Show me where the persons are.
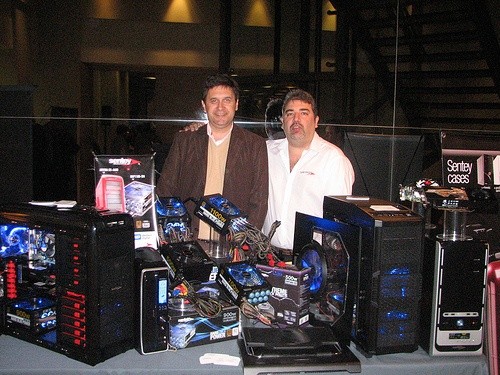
[110,124,128,155]
[324,126,372,196]
[155,73,269,251]
[265,98,287,140]
[179,89,355,262]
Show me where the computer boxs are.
[135,247,169,356]
[323,194,426,359]
[0,203,137,366]
[423,229,489,358]
